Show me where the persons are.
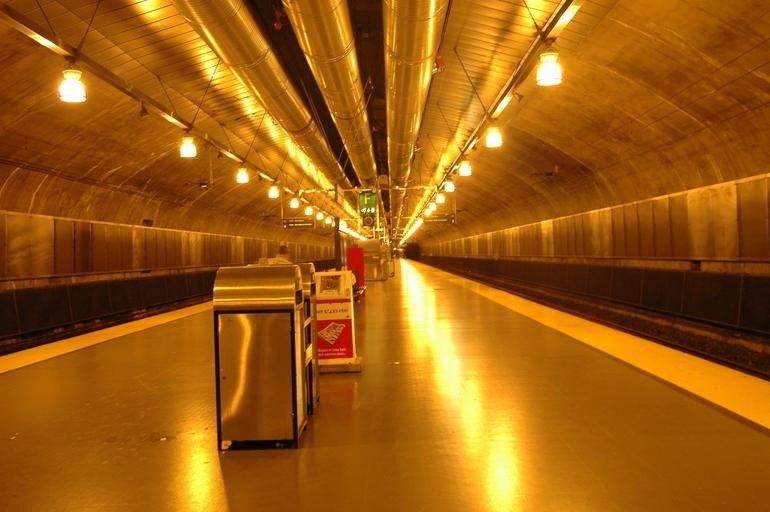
[267,246,293,264]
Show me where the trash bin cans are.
[213,263,320,451]
